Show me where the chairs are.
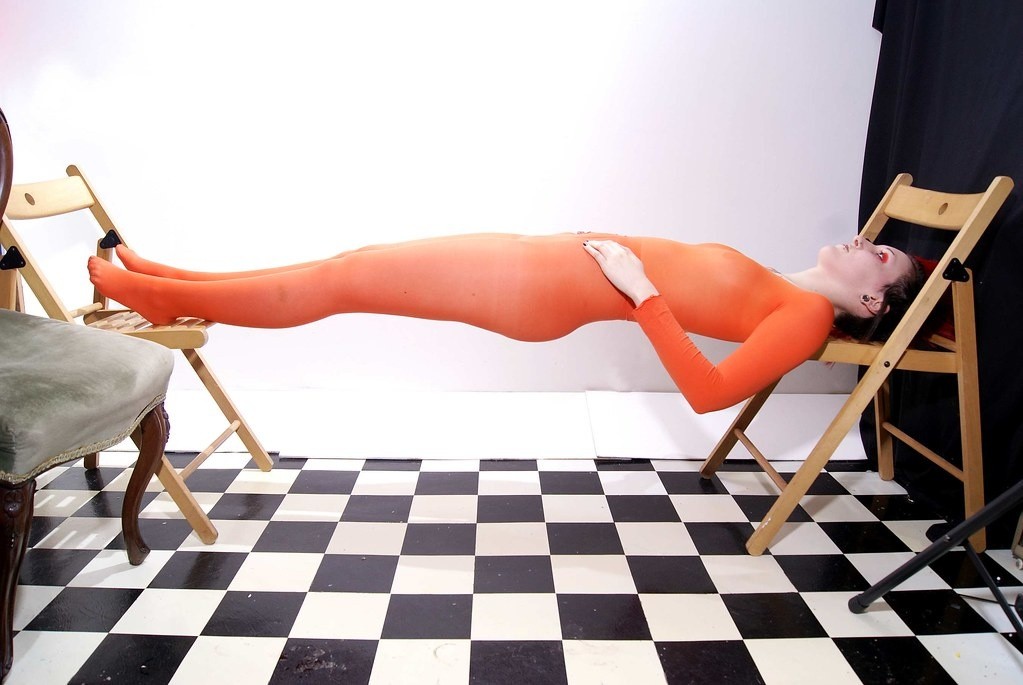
[0,106,173,685]
[702,174,1015,558]
[0,163,272,546]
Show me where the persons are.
[88,232,928,415]
